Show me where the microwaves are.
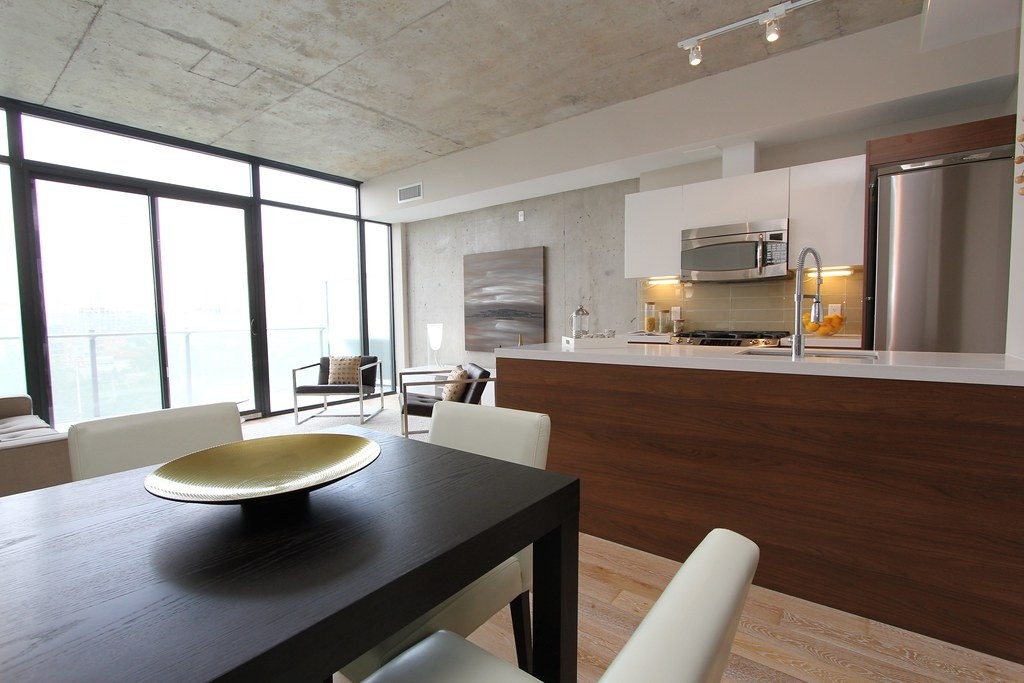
[680,217,789,283]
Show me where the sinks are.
[815,353,879,359]
[741,350,815,357]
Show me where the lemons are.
[801,313,844,335]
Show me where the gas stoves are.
[670,327,791,347]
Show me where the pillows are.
[441,365,468,401]
[328,356,361,384]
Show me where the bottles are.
[659,309,669,332]
[645,301,655,333]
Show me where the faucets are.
[787,245,824,358]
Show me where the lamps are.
[689,40,705,67]
[765,16,780,42]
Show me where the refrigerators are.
[871,141,1017,355]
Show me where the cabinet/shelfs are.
[625,153,866,278]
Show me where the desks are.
[0,424,580,683]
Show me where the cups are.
[673,318,684,333]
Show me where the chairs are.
[291,355,383,425]
[356,527,762,683]
[0,394,67,498]
[344,401,552,673]
[67,401,245,482]
[398,363,491,438]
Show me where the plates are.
[142,433,382,506]
